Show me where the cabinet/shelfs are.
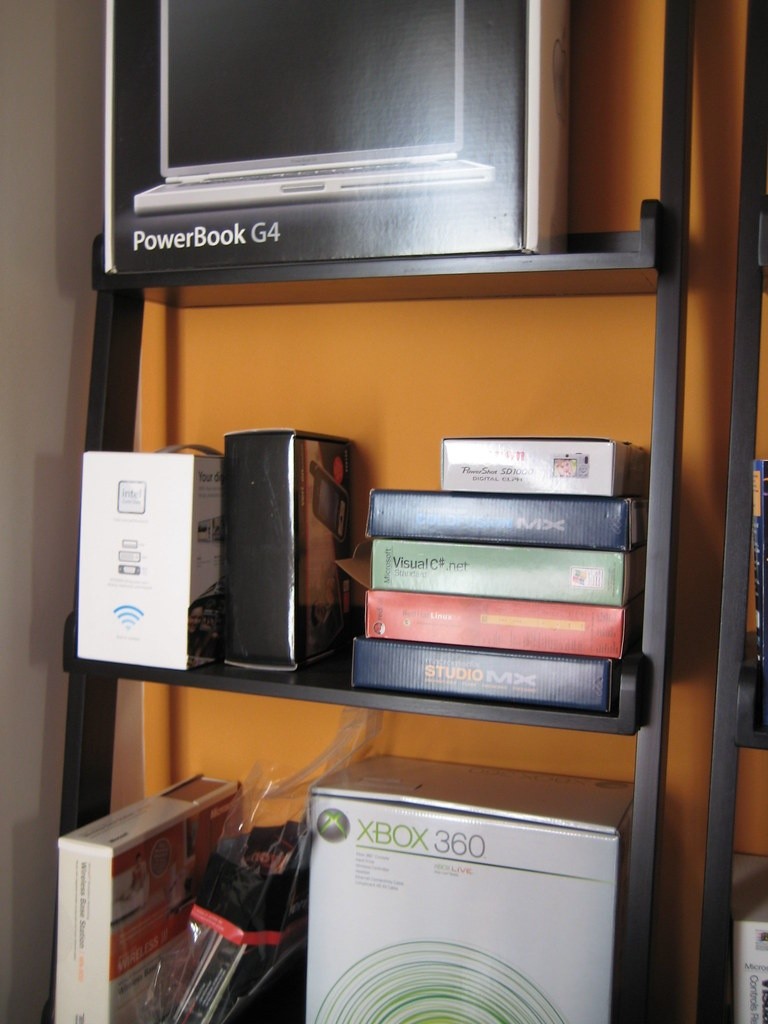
[43,1,695,1024]
[700,0,767,1024]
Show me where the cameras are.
[551,452,591,480]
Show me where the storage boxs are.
[306,754,633,1024]
[222,428,351,673]
[731,852,768,1024]
[77,446,223,670]
[352,436,650,714]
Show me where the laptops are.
[134,0,495,218]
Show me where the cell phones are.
[309,460,349,542]
